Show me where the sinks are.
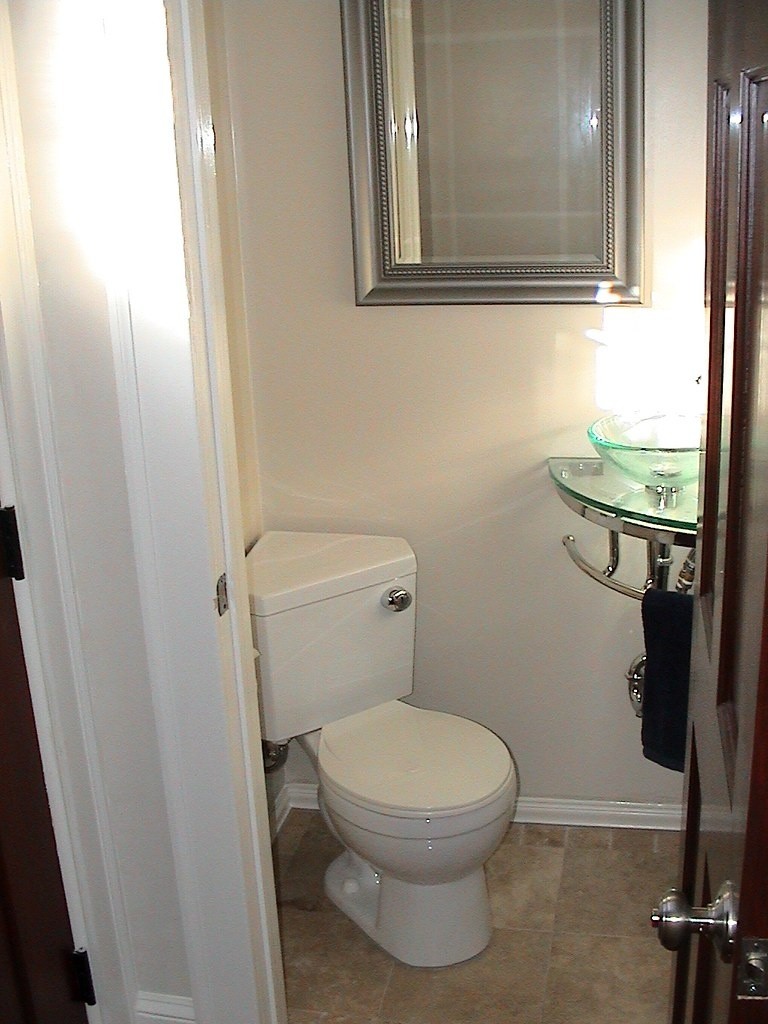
[585,407,706,490]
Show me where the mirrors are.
[339,0,645,308]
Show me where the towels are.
[639,586,696,774]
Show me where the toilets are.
[241,529,521,970]
